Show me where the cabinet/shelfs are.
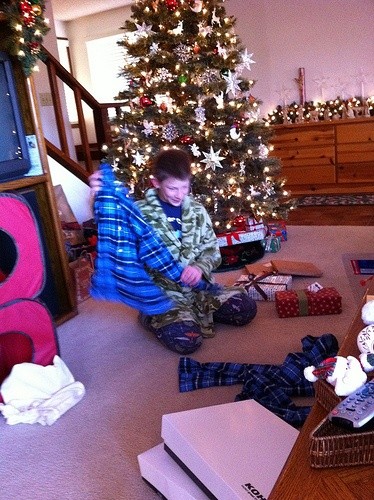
[272,118,374,198]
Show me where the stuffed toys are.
[303,355,367,398]
[357,300,374,374]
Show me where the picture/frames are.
[0,173,80,329]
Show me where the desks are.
[266,273,374,500]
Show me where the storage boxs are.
[160,399,301,500]
[275,287,343,318]
[233,274,293,303]
[217,224,266,247]
[137,441,209,500]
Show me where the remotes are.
[328,376,374,428]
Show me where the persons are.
[89,150,257,356]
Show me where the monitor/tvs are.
[0,50,32,182]
